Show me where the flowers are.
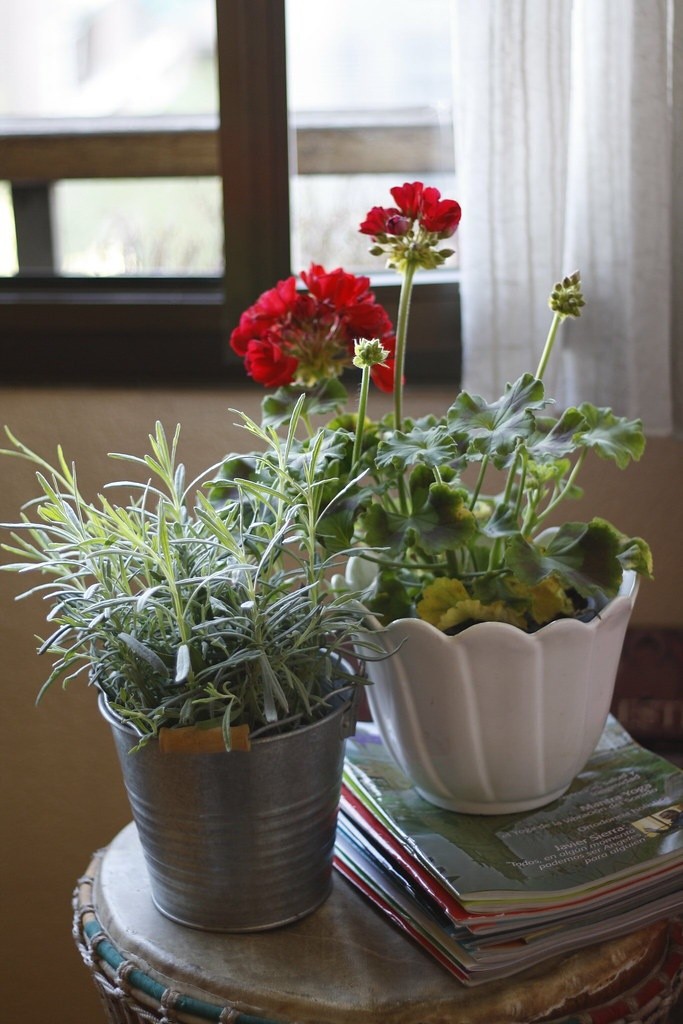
[202,176,658,625]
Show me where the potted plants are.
[0,388,411,933]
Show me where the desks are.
[69,821,683,1024]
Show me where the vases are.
[328,527,638,816]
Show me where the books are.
[331,712,683,988]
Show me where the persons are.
[644,810,683,834]
